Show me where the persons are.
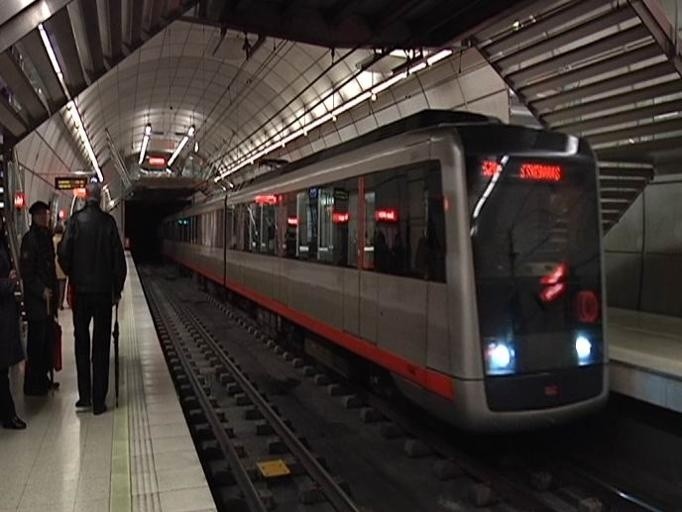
[62,219,73,308]
[51,224,66,312]
[18,200,59,397]
[57,181,127,416]
[0,214,27,431]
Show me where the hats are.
[29,201,50,213]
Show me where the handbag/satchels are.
[53,321,61,371]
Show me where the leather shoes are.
[3,417,26,429]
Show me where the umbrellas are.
[111,302,122,410]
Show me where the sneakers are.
[76,400,91,406]
[94,404,106,414]
[24,376,59,395]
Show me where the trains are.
[160,107,613,433]
